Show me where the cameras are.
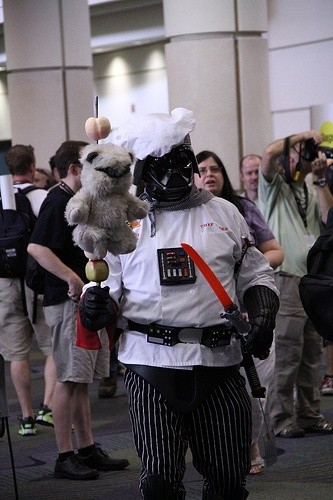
[305,138,333,162]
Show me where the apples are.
[85,260,109,281]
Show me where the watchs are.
[313,177,326,187]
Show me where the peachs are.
[85,117,111,141]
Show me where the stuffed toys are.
[65,141,151,264]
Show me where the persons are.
[83,107,283,500]
[23,142,131,482]
[32,169,55,191]
[192,149,284,476]
[236,154,261,205]
[0,144,75,437]
[258,130,333,438]
[48,149,63,188]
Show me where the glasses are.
[198,166,223,174]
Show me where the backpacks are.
[0,185,43,277]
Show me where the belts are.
[125,320,237,343]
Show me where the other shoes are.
[76,448,130,471]
[54,455,100,480]
[320,375,333,395]
[276,424,305,439]
[309,419,333,432]
[96,380,118,399]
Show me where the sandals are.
[250,439,265,474]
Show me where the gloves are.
[243,326,275,360]
[83,284,113,323]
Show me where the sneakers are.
[37,404,76,432]
[17,415,38,437]
